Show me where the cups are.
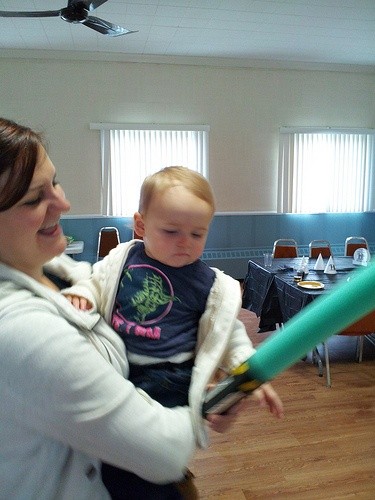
[263,254,273,267]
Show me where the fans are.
[0,0,139,38]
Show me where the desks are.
[241,257,375,376]
[64,241,84,260]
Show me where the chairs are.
[309,240,332,258]
[97,227,120,262]
[345,237,369,257]
[312,306,375,387]
[272,239,298,258]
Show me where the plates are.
[297,280,325,289]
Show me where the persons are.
[0,118,246,500]
[59,166,283,500]
